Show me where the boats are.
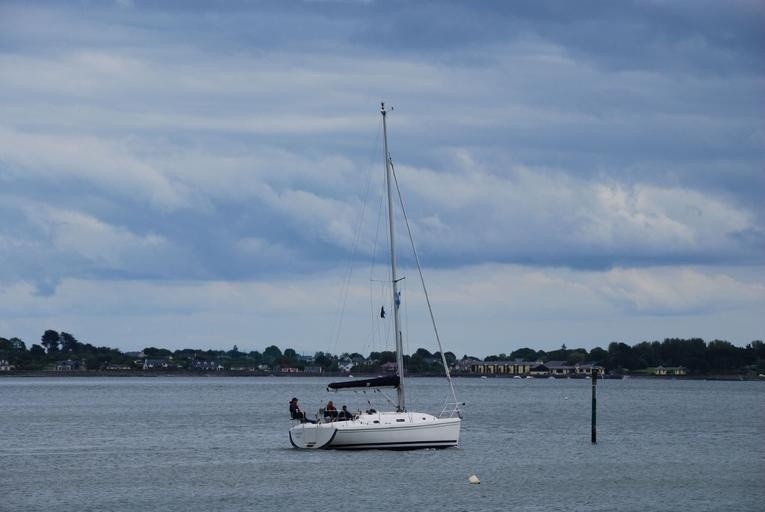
[327,374,401,390]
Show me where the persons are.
[336,404,353,420]
[323,400,338,421]
[288,397,316,424]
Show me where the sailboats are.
[285,98,469,452]
